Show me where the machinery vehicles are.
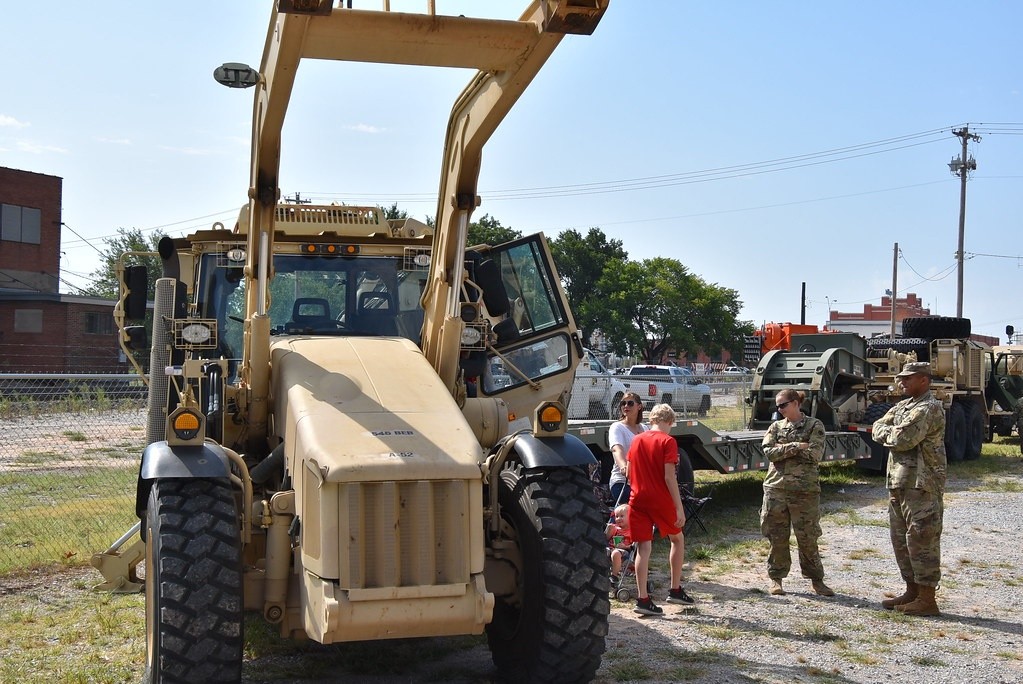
[569,316,1023,518]
[91,0,614,684]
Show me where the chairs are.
[293,298,337,328]
[359,292,397,336]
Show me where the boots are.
[882,582,940,616]
[771,578,784,594]
[812,579,835,596]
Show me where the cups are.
[612,535,623,548]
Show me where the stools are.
[677,481,721,535]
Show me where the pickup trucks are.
[618,364,713,418]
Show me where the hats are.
[896,362,932,377]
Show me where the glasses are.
[776,400,793,411]
[620,400,639,407]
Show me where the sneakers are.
[633,595,663,616]
[666,585,694,605]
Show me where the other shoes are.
[609,573,622,583]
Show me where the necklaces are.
[628,423,640,434]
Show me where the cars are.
[722,366,748,374]
[567,348,628,420]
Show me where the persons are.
[626,403,694,614]
[872,362,947,617]
[608,393,652,574]
[758,390,835,596]
[604,504,633,583]
